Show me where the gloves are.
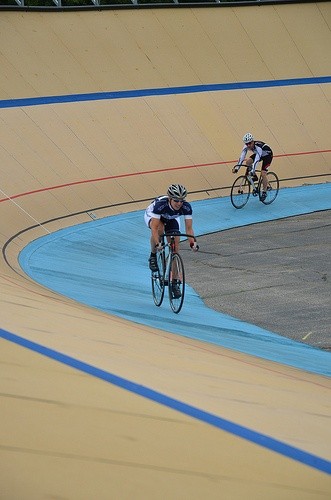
[155,243,164,251]
[190,241,199,253]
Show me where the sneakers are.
[173,285,181,298]
[148,255,159,272]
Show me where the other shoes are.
[260,192,267,201]
[248,176,258,184]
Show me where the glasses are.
[245,141,253,145]
[172,199,186,203]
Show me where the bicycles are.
[230,164,280,210]
[151,222,200,313]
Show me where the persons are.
[231,134,274,201]
[145,184,199,299]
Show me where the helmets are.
[242,133,254,144]
[167,184,188,201]
